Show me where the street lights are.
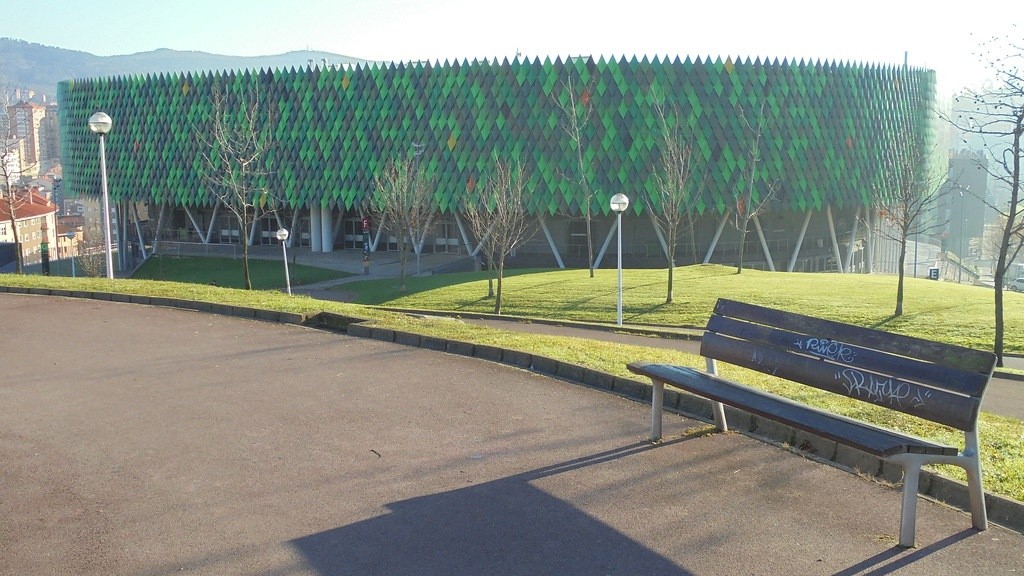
[88,112,112,277]
[610,192,629,325]
[276,228,291,296]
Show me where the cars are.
[1008,278,1024,292]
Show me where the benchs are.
[627,297,997,548]
